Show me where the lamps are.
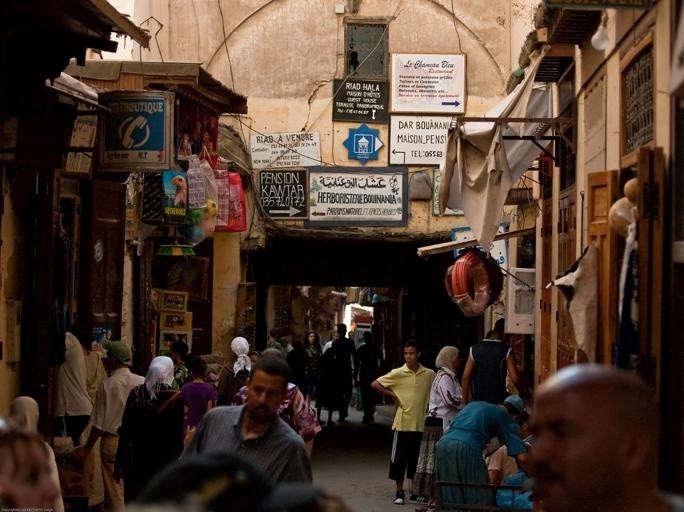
[590,9,611,51]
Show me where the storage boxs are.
[159,312,193,330]
[160,289,189,312]
[158,330,192,354]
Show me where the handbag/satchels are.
[140,153,247,232]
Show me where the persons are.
[371,318,684,510]
[176,111,216,161]
[1,323,343,512]
[303,324,384,425]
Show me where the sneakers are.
[409,495,428,504]
[394,490,404,504]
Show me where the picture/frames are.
[304,166,408,226]
[388,113,457,167]
[451,223,507,268]
[387,51,467,115]
[260,167,311,220]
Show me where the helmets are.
[103,339,128,361]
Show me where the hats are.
[114,347,117,348]
[506,395,524,414]
